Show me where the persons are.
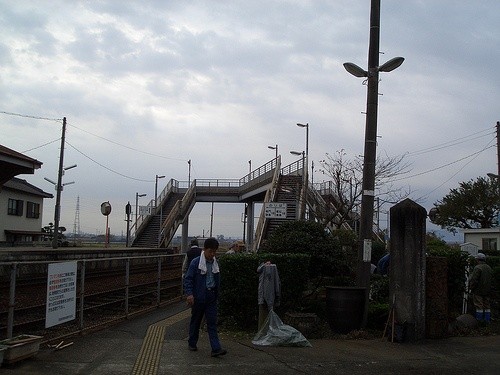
[182,238,203,306]
[226,241,246,254]
[469,253,493,321]
[184,238,228,357]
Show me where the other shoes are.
[188,344,198,352]
[210,349,227,357]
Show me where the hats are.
[475,253,486,261]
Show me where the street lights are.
[154,175,165,206]
[135,192,146,231]
[269,145,277,170]
[343,57,405,335]
[297,122,309,186]
[290,150,305,184]
